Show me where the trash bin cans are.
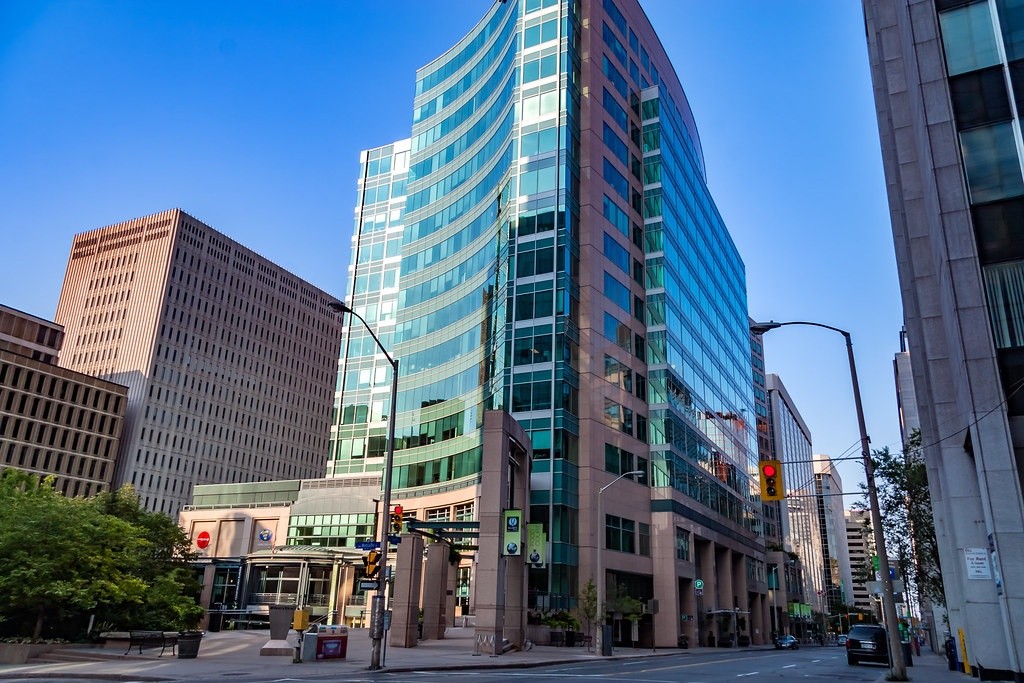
[901,640,913,667]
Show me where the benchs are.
[549,632,565,647]
[576,633,593,648]
[124,630,178,658]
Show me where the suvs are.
[846,622,893,667]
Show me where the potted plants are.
[565,618,580,647]
[173,605,204,658]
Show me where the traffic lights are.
[360,554,369,578]
[393,506,403,531]
[369,551,383,575]
[759,460,784,501]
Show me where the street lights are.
[596,471,644,655]
[327,299,399,669]
[753,322,912,682]
[821,588,836,625]
[773,560,794,637]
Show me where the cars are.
[775,635,799,650]
[838,637,847,646]
